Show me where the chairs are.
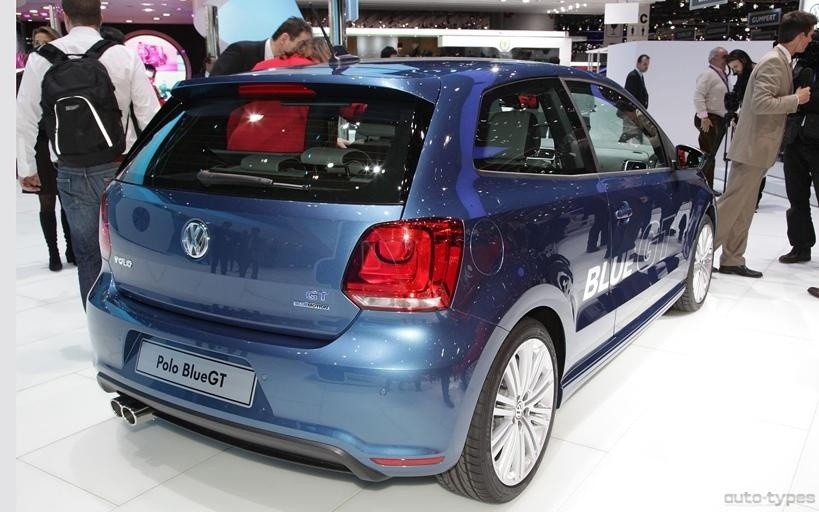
[348,122,394,160]
[476,113,559,176]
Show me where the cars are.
[85,56,718,505]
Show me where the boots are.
[60,210,79,267]
[39,211,62,271]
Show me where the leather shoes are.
[712,189,721,197]
[808,287,819,298]
[779,245,811,263]
[712,263,763,277]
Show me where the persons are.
[16,0,161,315]
[100,23,126,47]
[379,46,398,58]
[692,46,732,199]
[203,220,276,279]
[16,26,77,272]
[616,53,652,144]
[777,27,818,264]
[222,39,369,154]
[725,48,768,215]
[207,16,365,153]
[583,180,681,263]
[713,9,819,279]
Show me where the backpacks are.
[34,39,134,167]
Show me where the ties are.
[642,74,645,89]
